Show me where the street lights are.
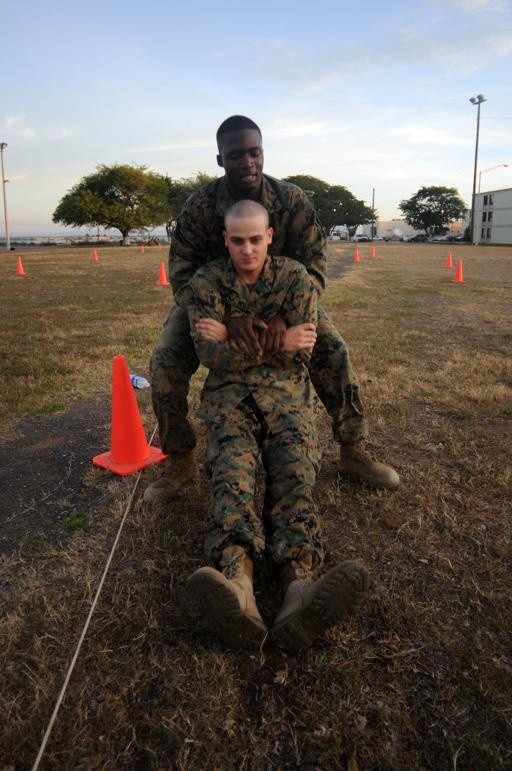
[477,164,509,192]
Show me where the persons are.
[141,114,401,506]
[186,198,371,657]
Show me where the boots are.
[143,447,198,504]
[273,560,369,654]
[187,544,269,645]
[337,442,401,491]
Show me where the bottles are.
[129,374,150,389]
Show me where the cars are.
[454,234,464,242]
[328,229,386,242]
[431,235,450,241]
[402,233,429,245]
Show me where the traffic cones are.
[139,244,146,254]
[449,257,465,284]
[368,244,377,257]
[15,256,27,277]
[446,250,454,270]
[157,244,162,251]
[351,247,360,264]
[91,249,100,262]
[154,260,171,287]
[90,351,168,477]
